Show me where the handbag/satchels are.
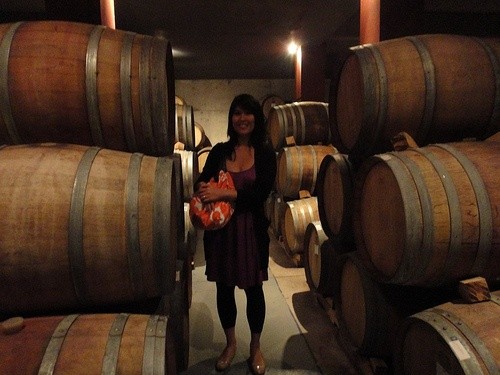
[189,143,237,230]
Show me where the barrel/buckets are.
[0,19,215,375]
[256,33,499,374]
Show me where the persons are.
[194,94,277,375]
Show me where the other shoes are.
[216,340,237,371]
[250,342,266,374]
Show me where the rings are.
[204,195,205,197]
